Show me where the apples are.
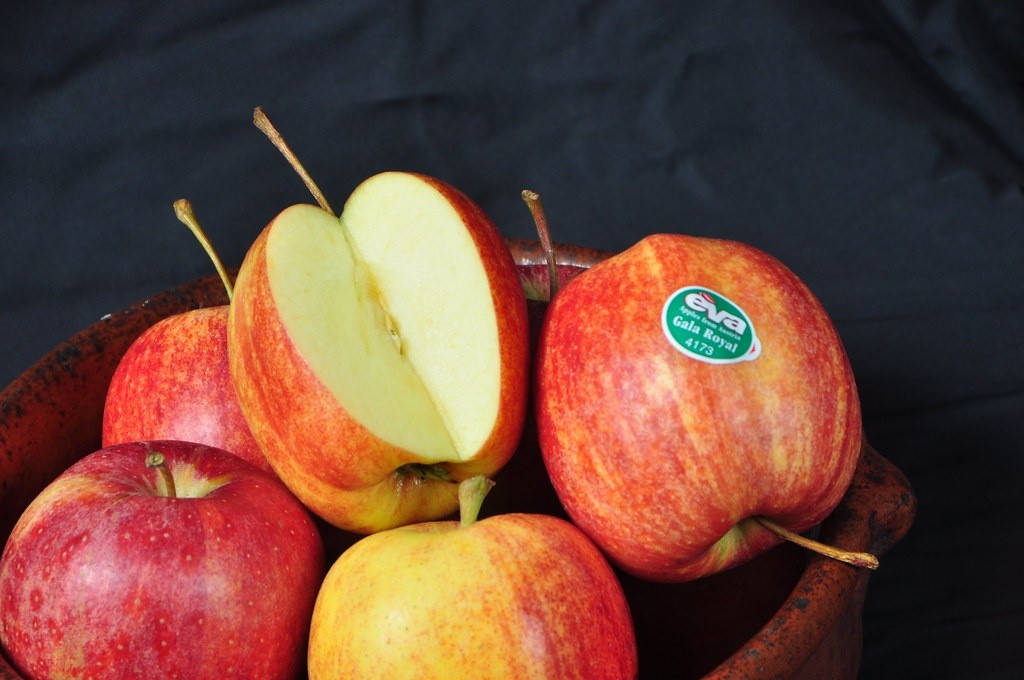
[0,107,881,680]
